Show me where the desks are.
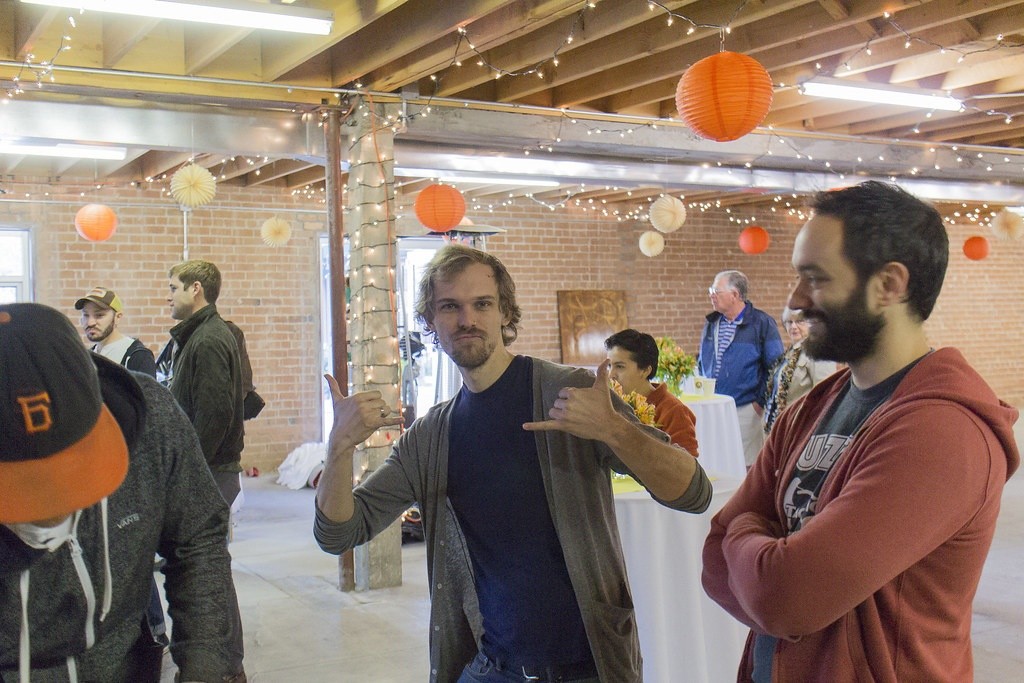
[671,394,748,476]
[615,475,751,683]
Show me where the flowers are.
[605,378,655,488]
[653,336,695,398]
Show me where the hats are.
[0,302,129,525]
[75,286,122,312]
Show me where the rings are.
[380,408,385,418]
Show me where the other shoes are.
[173,667,248,683]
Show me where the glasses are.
[708,286,735,295]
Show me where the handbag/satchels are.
[400,330,424,366]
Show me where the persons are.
[0,302,247,683]
[701,181,1020,683]
[74,260,266,655]
[697,270,835,472]
[604,329,700,458]
[313,244,713,683]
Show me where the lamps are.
[797,75,962,112]
[20,0,333,37]
